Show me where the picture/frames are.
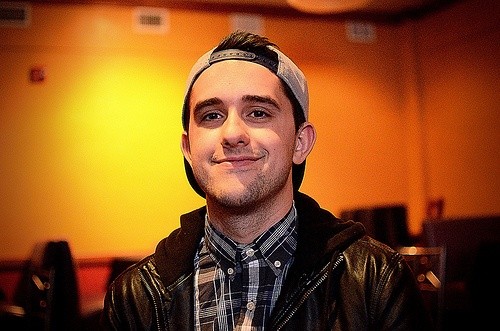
[390,246,446,292]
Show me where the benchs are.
[0,238,108,331]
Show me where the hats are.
[182,45,309,199]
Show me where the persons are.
[99,33,427,331]
